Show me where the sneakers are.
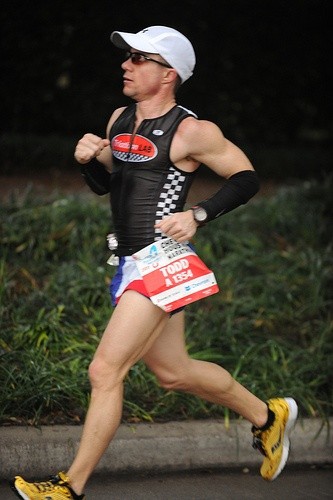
[11,470,84,500]
[252,396,298,481]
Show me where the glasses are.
[123,52,173,67]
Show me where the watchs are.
[190,205,207,226]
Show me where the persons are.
[0,26,298,500]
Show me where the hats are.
[109,25,196,83]
